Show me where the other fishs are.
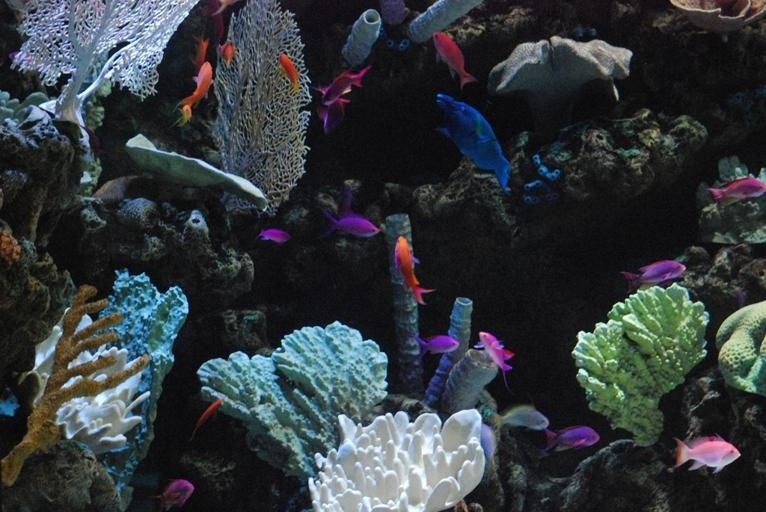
[169,0,237,135]
